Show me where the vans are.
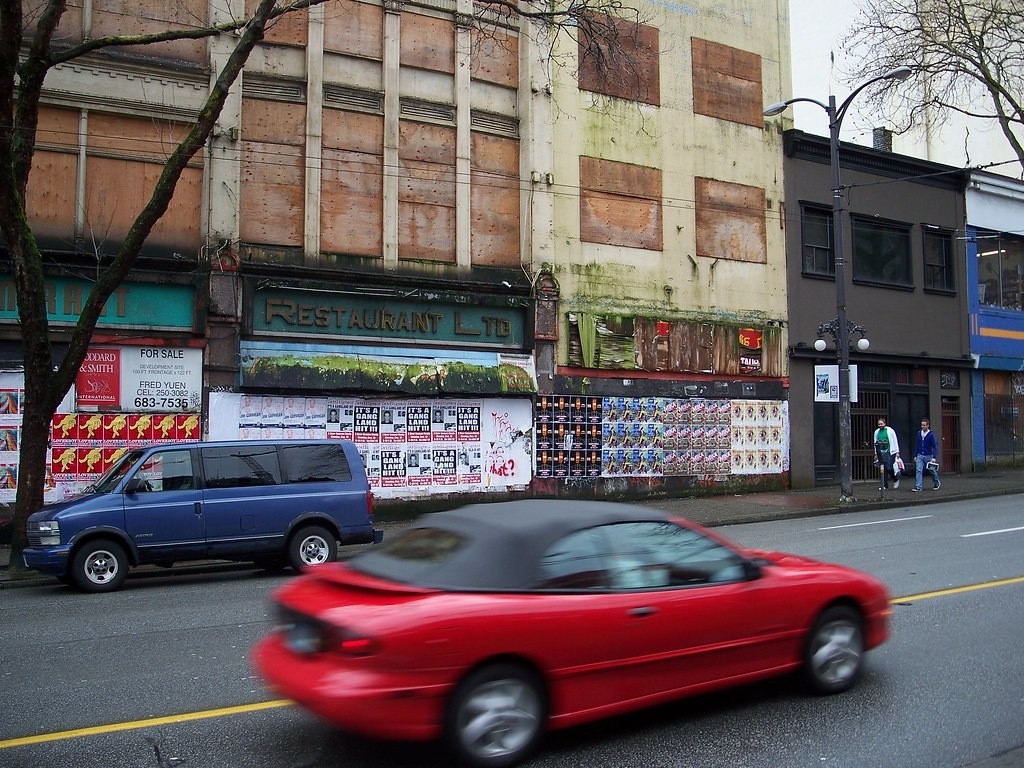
[23,438,384,594]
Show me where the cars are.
[256,498,891,768]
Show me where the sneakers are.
[893,479,899,488]
[911,487,923,492]
[932,479,941,490]
[878,486,887,490]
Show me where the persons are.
[911,418,942,492]
[874,418,902,490]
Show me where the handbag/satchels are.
[893,456,905,476]
[925,461,939,471]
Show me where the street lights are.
[762,65,911,502]
